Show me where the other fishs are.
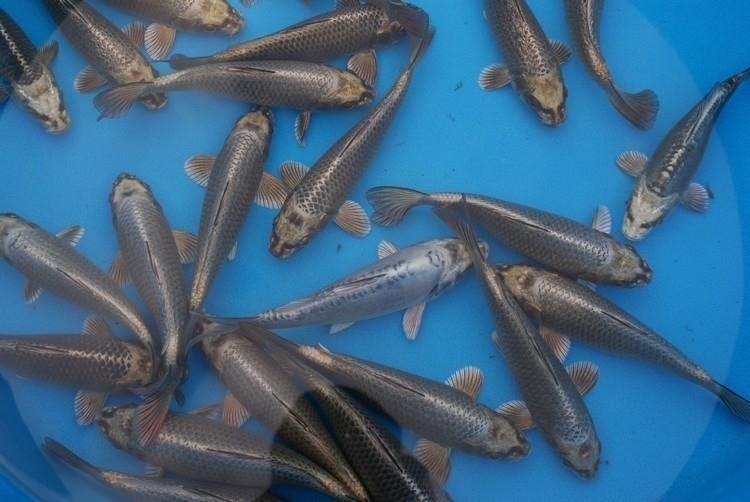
[1,1,746,502]
